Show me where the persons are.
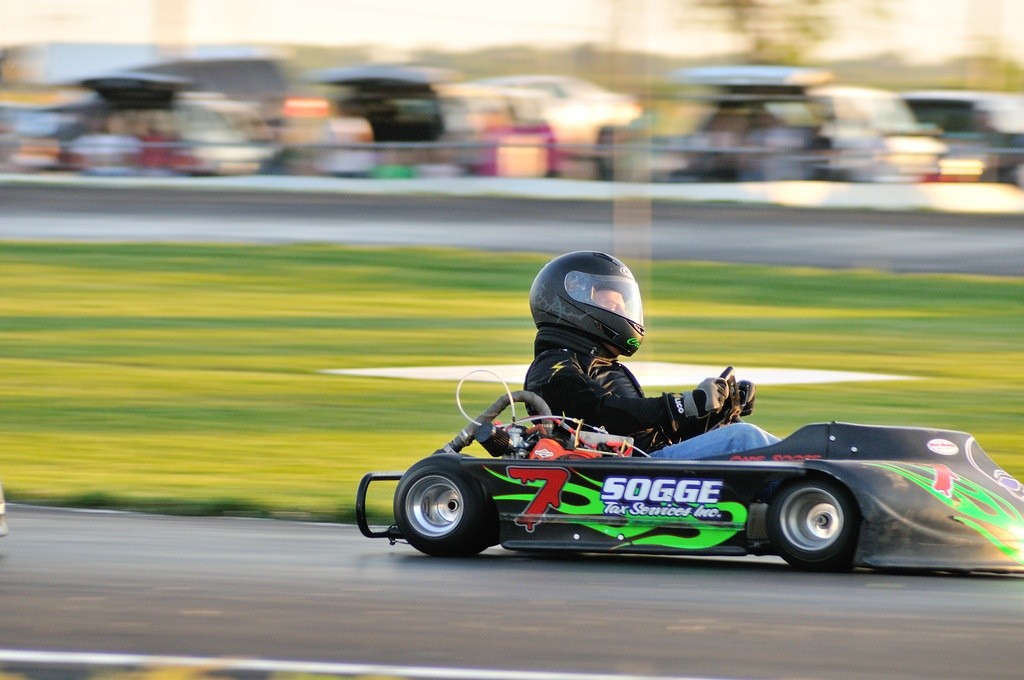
[523,249,785,460]
[0,100,1024,191]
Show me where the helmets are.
[529,250,646,354]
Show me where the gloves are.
[731,379,757,417]
[680,376,728,418]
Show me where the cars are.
[0,48,1024,186]
